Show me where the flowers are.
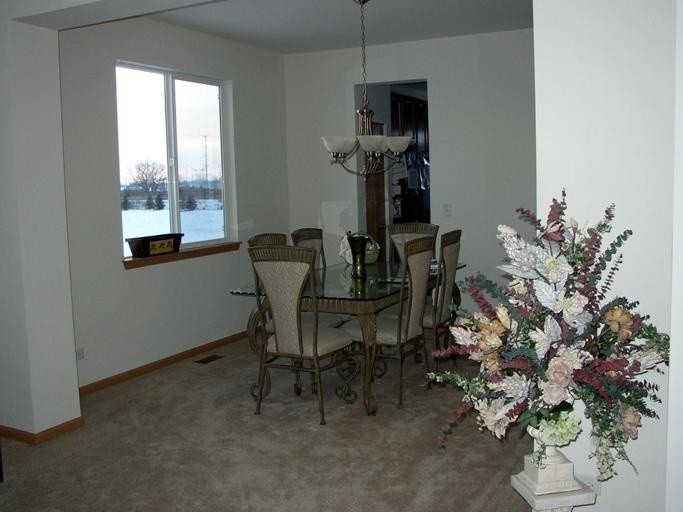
[418,187,670,483]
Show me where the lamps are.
[320,1,411,178]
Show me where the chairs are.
[245,231,345,395]
[384,223,442,372]
[288,227,358,327]
[376,228,464,388]
[338,234,437,409]
[245,243,358,425]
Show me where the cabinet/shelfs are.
[390,92,430,224]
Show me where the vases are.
[124,233,184,258]
[512,425,583,498]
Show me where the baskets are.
[350,232,380,265]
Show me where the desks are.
[230,257,468,417]
